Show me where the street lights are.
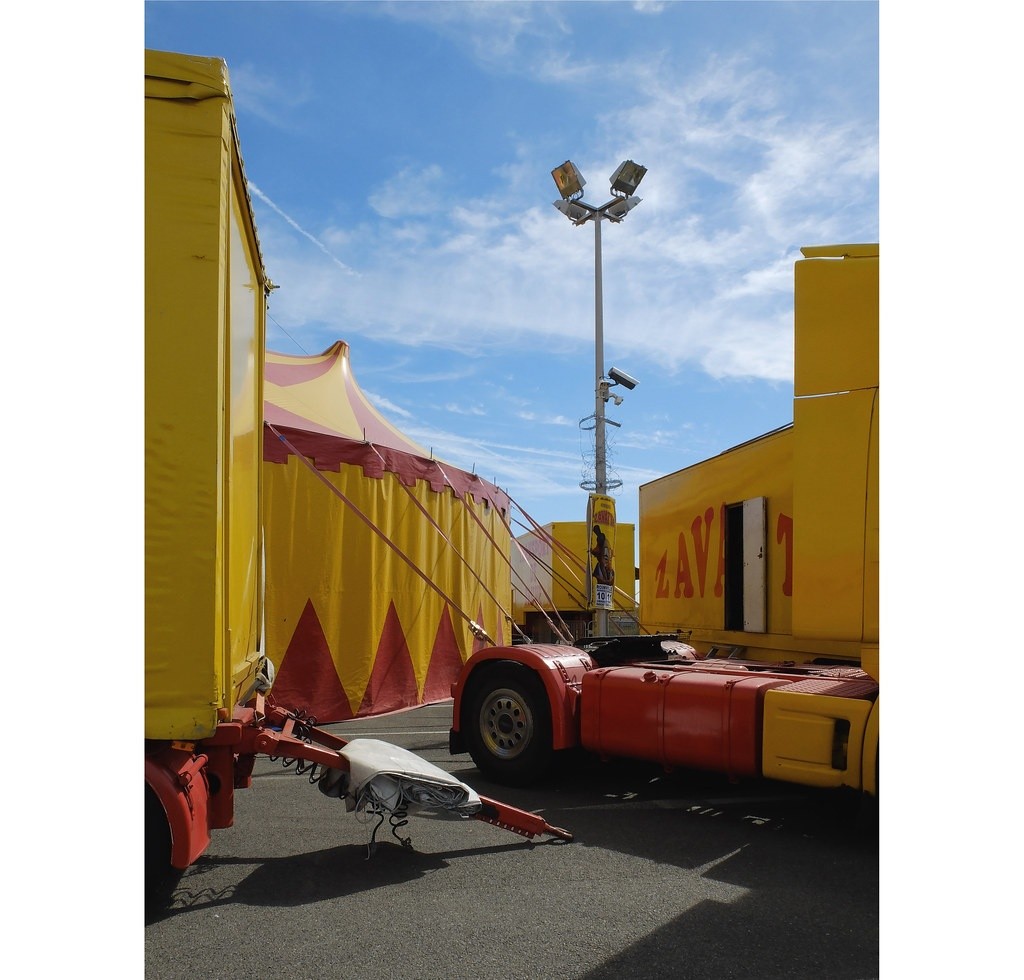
[551,159,648,645]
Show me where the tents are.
[261,340,513,729]
